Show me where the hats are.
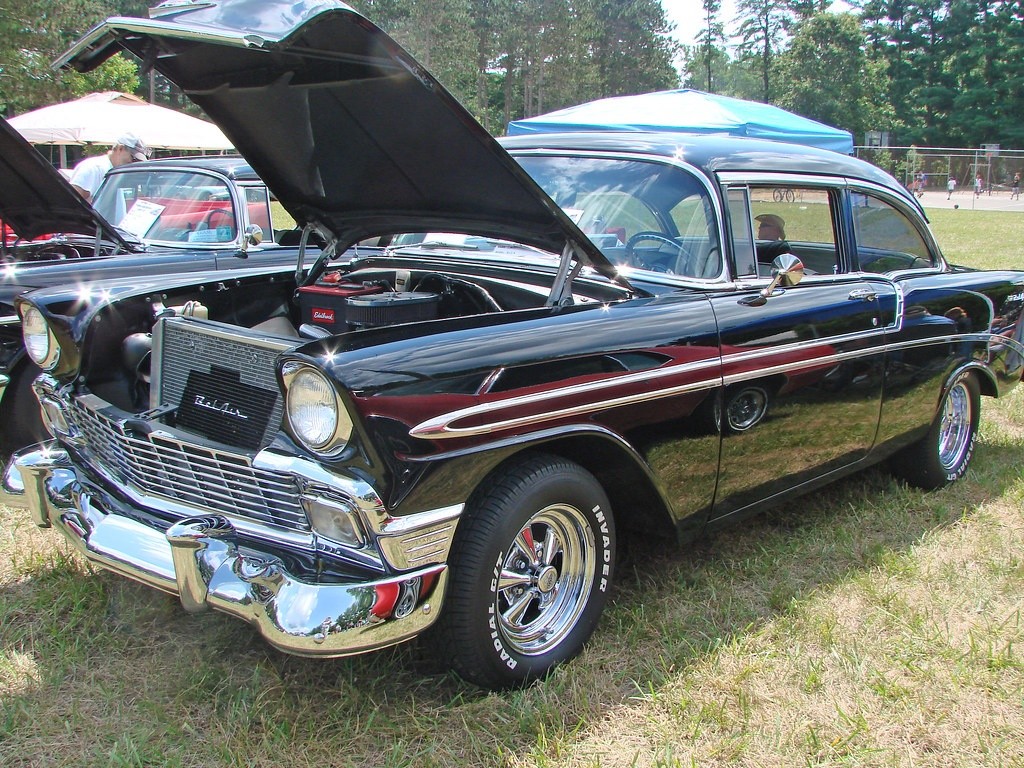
[754,213,785,229]
[117,132,152,162]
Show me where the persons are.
[975,175,982,197]
[915,169,927,198]
[754,213,788,242]
[948,176,957,201]
[69,131,147,228]
[1010,174,1020,201]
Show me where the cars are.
[1,175,269,248]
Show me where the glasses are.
[759,222,774,227]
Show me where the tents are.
[509,88,854,156]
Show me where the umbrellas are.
[7,91,235,151]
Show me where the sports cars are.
[0,113,626,387]
[0,0,1024,689]
